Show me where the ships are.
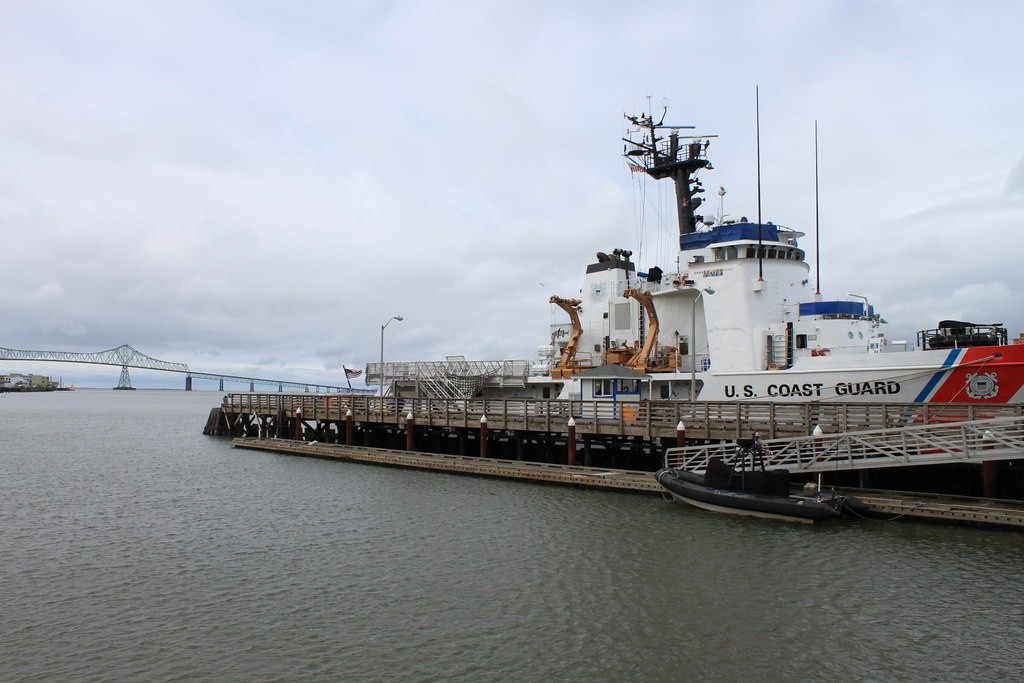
[363,87,1024,455]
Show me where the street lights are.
[379,314,404,397]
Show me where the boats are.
[654,433,869,528]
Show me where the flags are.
[627,161,647,173]
[344,368,363,379]
